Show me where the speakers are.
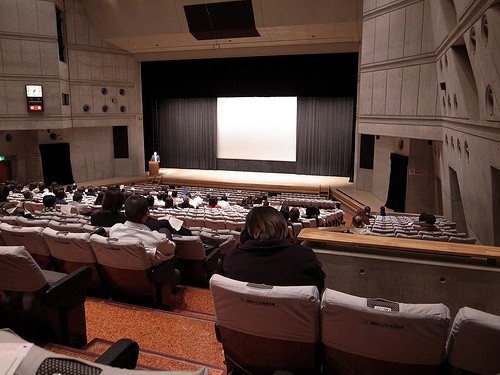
[428,140,432,145]
[440,82,446,90]
[376,135,380,139]
[47,129,50,133]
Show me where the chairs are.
[0,182,500,375]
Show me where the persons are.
[344,206,376,233]
[331,203,346,224]
[223,206,326,293]
[412,214,441,231]
[375,206,388,216]
[413,213,428,225]
[151,152,160,162]
[0,179,320,295]
